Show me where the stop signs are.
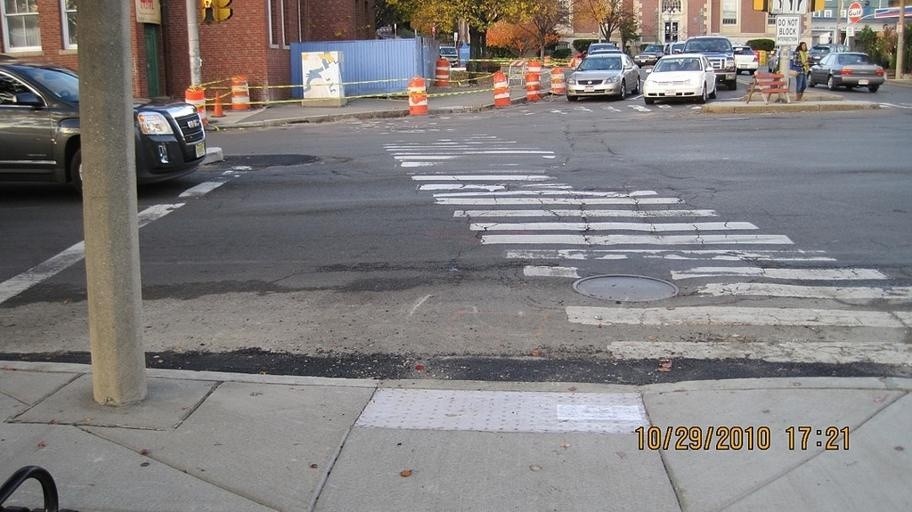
[848,2,863,24]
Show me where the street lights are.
[394,23,397,37]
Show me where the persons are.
[790,40,811,101]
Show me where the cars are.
[664,40,685,55]
[768,49,795,72]
[642,53,717,104]
[805,52,887,92]
[566,53,642,101]
[634,43,670,70]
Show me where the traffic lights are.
[213,0,232,22]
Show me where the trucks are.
[439,46,459,66]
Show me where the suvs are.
[673,35,739,90]
[590,48,624,55]
[732,45,759,75]
[588,41,617,56]
[807,43,849,66]
[1,62,206,187]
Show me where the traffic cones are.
[211,90,227,120]
